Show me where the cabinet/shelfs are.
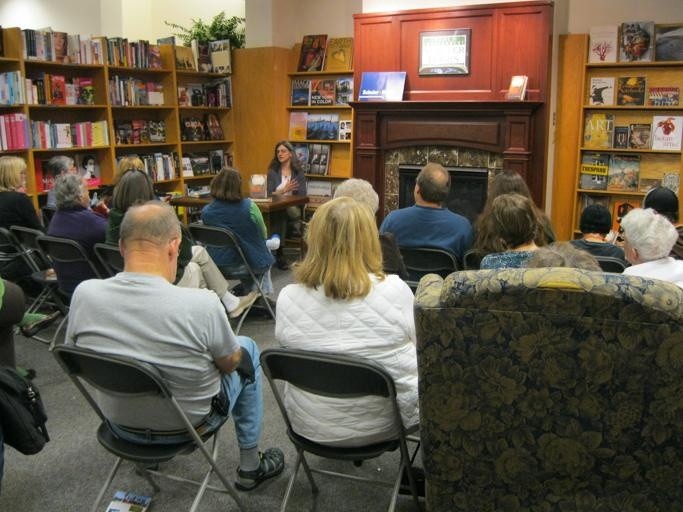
[287,38,354,181]
[0,27,236,233]
[550,34,682,246]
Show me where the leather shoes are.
[228,291,256,321]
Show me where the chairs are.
[588,254,628,275]
[91,237,130,284]
[395,246,460,285]
[49,340,254,511]
[257,344,425,511]
[0,224,44,341]
[462,249,494,272]
[418,268,682,512]
[189,221,278,338]
[28,234,104,348]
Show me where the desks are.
[167,186,314,272]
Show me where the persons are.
[479,193,544,270]
[93,154,147,216]
[81,156,96,182]
[379,162,474,272]
[268,140,308,271]
[0,277,61,380]
[474,173,555,254]
[275,197,421,449]
[333,177,378,221]
[47,156,81,211]
[622,206,682,290]
[63,201,285,493]
[0,156,46,278]
[643,187,683,261]
[568,205,632,275]
[105,169,258,319]
[200,167,280,300]
[522,240,602,274]
[45,175,114,296]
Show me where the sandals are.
[233,447,286,490]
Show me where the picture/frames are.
[418,28,472,76]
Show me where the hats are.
[642,184,680,214]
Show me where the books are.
[25,70,96,104]
[178,76,233,107]
[312,78,337,106]
[191,39,232,75]
[506,75,529,101]
[23,27,104,64]
[578,152,610,190]
[576,194,610,230]
[74,153,101,187]
[249,173,268,200]
[620,20,656,62]
[175,45,195,71]
[652,115,683,151]
[105,37,163,68]
[0,113,31,151]
[0,70,25,105]
[34,153,44,192]
[614,125,629,149]
[638,177,663,193]
[629,123,652,149]
[617,75,646,107]
[292,143,309,174]
[185,183,210,199]
[179,112,225,141]
[288,112,308,140]
[290,79,312,106]
[607,153,640,192]
[583,112,614,149]
[296,34,328,72]
[612,199,641,247]
[110,75,166,105]
[116,150,181,181]
[661,165,679,194]
[157,36,176,68]
[649,86,680,107]
[335,77,354,106]
[247,196,272,203]
[183,148,234,177]
[637,154,679,195]
[654,24,682,61]
[587,24,621,63]
[307,144,332,176]
[32,119,109,149]
[589,76,615,107]
[324,37,355,71]
[113,118,167,145]
